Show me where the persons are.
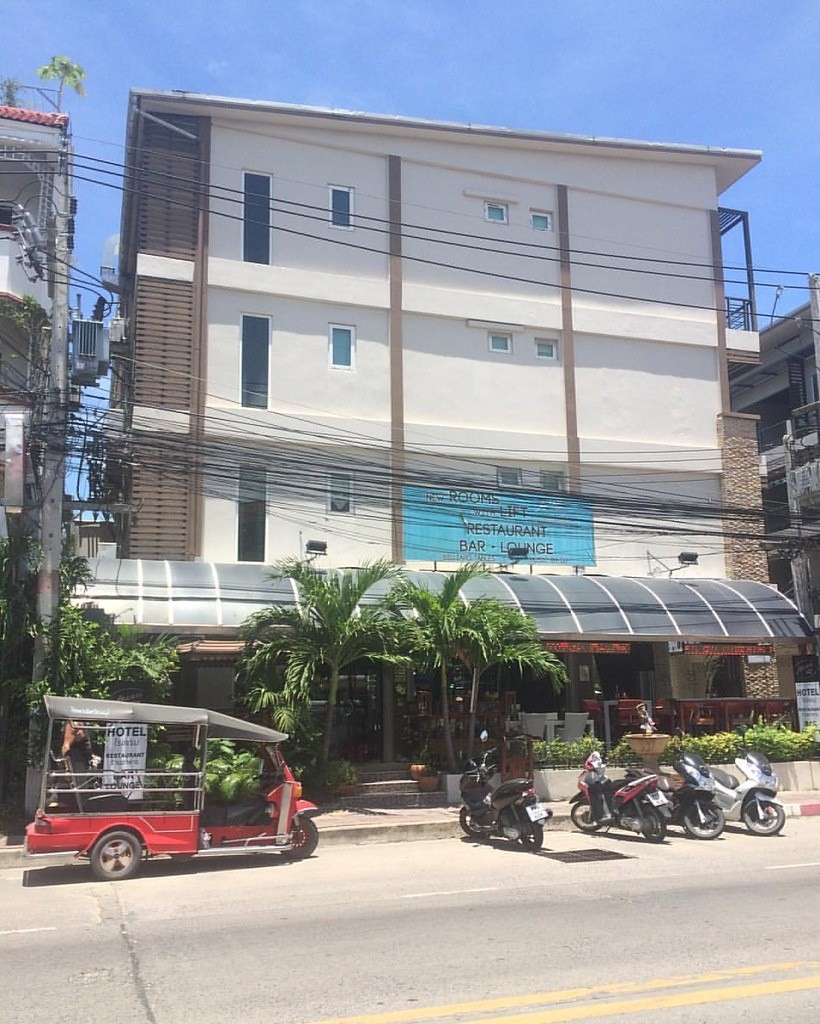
[61,721,92,805]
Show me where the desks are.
[669,697,799,738]
[601,701,652,740]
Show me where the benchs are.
[185,764,257,827]
[51,754,127,812]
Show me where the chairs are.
[647,698,665,729]
[450,695,482,732]
[417,689,450,733]
[581,699,606,738]
[489,692,517,732]
[613,700,645,738]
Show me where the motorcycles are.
[458,728,554,849]
[706,723,787,837]
[624,727,727,842]
[568,747,673,844]
[23,693,319,883]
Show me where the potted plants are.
[418,761,439,792]
[410,734,431,781]
[336,758,357,796]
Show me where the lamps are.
[305,540,329,564]
[663,552,700,578]
[509,546,529,558]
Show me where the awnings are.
[61,557,819,645]
